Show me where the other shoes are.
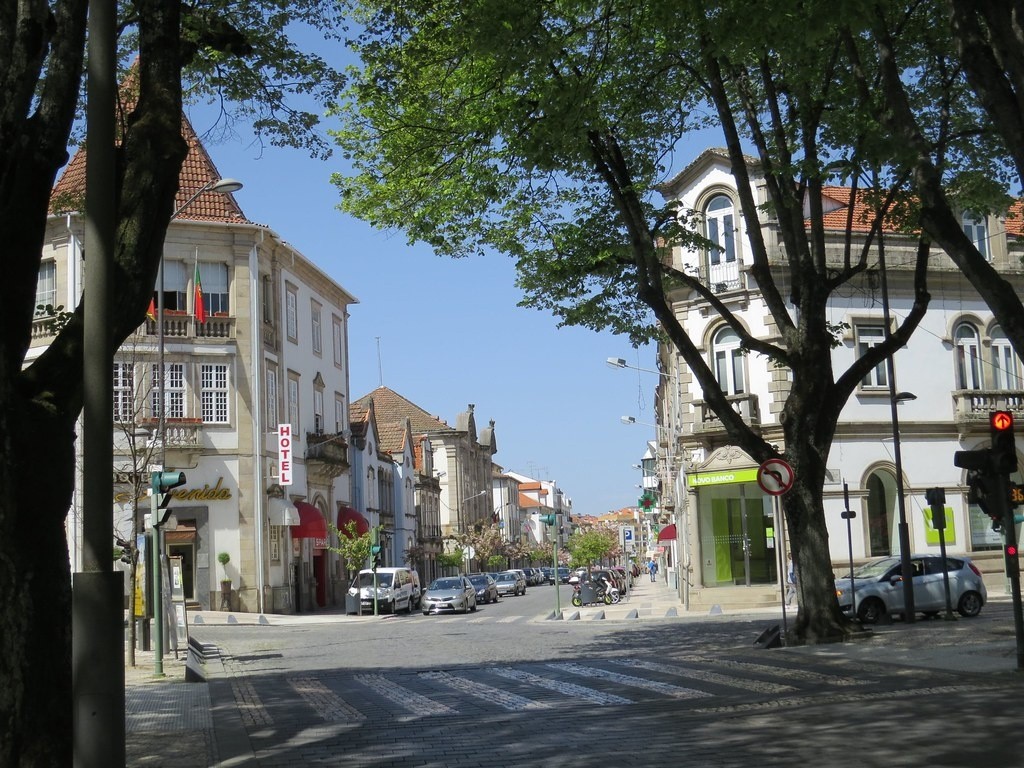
[785,605,790,609]
[651,581,656,582]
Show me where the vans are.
[347,567,414,614]
[411,571,421,609]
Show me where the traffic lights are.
[153,471,186,526]
[1009,484,1024,505]
[371,546,381,571]
[1004,544,1020,577]
[954,449,999,519]
[540,515,555,542]
[989,410,1018,472]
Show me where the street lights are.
[156,179,243,653]
[827,158,916,622]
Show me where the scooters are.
[572,574,621,606]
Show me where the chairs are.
[912,564,930,577]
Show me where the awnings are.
[337,507,369,540]
[658,524,676,541]
[688,468,759,487]
[291,501,327,538]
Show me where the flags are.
[147,299,156,323]
[193,263,206,325]
[491,512,499,523]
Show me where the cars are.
[575,568,587,577]
[550,568,570,585]
[834,554,986,623]
[485,568,544,587]
[494,573,526,597]
[421,577,476,615]
[592,564,640,594]
[465,575,497,604]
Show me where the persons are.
[785,553,798,609]
[633,565,637,578]
[648,560,658,582]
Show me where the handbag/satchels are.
[790,573,797,583]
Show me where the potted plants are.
[218,552,233,612]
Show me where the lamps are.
[634,484,661,493]
[620,415,673,432]
[632,464,658,473]
[606,357,676,381]
